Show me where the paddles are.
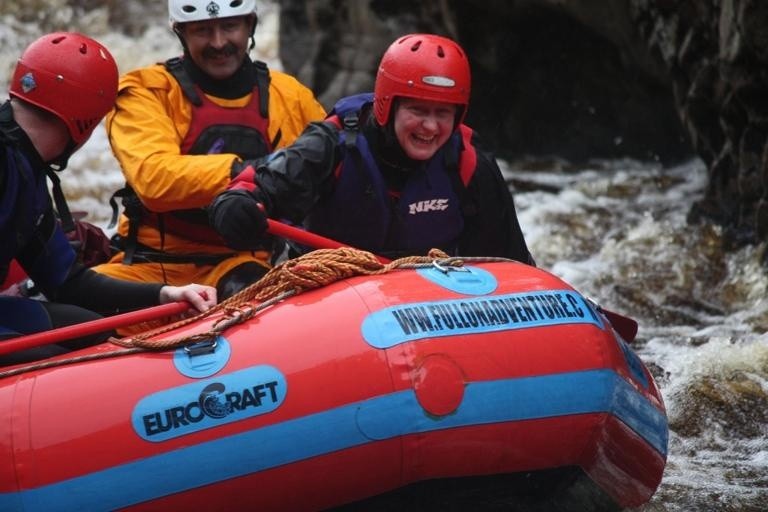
[254,202,638,345]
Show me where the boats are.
[0,250,670,511]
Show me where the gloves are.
[230,146,290,180]
[212,197,269,247]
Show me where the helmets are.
[373,32,472,136]
[9,31,119,155]
[168,1,262,52]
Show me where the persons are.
[1,31,219,366]
[86,1,329,336]
[206,31,539,267]
